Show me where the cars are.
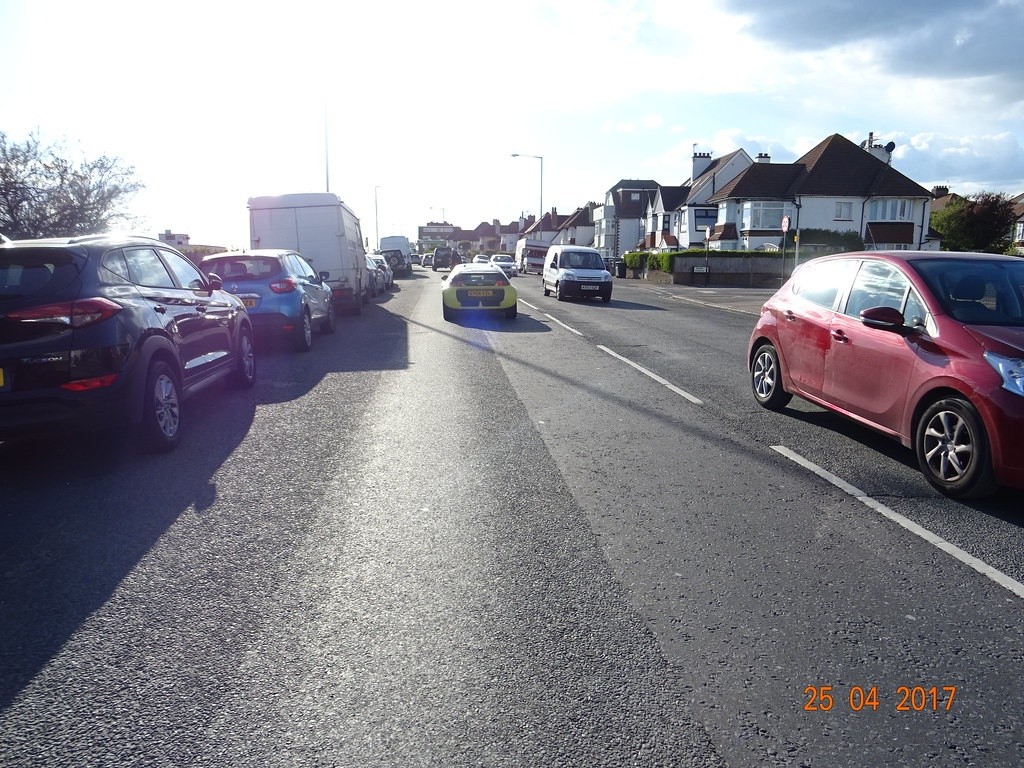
[364,235,467,298]
[441,262,518,321]
[197,248,335,352]
[746,249,1024,499]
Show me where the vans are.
[542,244,613,303]
[431,245,453,272]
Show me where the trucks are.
[246,191,370,312]
[514,237,552,276]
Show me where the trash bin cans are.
[615,262,626,278]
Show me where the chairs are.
[950,274,994,326]
[18,261,52,290]
[997,280,1024,325]
[582,255,593,267]
[230,262,247,278]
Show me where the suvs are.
[471,255,490,264]
[489,254,519,277]
[0,230,258,455]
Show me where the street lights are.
[512,153,543,243]
[375,186,381,252]
